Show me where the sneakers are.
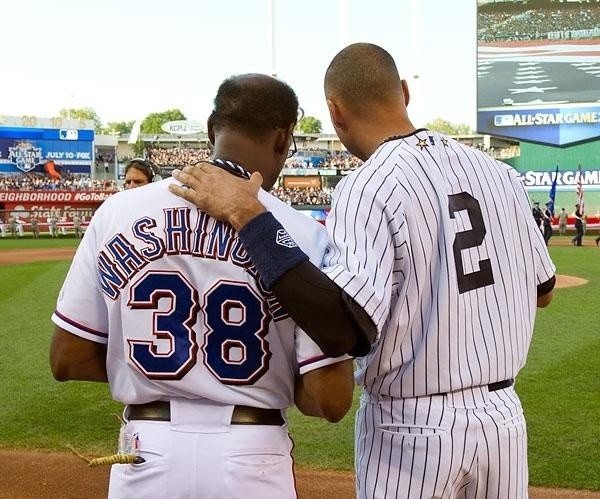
[569,238,600,248]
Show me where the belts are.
[428,378,515,397]
[125,398,288,427]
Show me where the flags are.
[576,163,584,216]
[548,165,559,220]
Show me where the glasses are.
[272,121,298,160]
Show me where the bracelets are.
[233,210,311,294]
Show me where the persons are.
[557,208,569,237]
[45,71,355,499]
[571,204,587,246]
[168,42,558,497]
[1,211,84,240]
[145,144,211,167]
[270,184,336,206]
[470,143,495,155]
[284,146,365,169]
[543,201,555,246]
[95,150,116,174]
[477,0,600,42]
[531,201,544,234]
[2,174,120,192]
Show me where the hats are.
[534,202,550,206]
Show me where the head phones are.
[133,158,163,183]
